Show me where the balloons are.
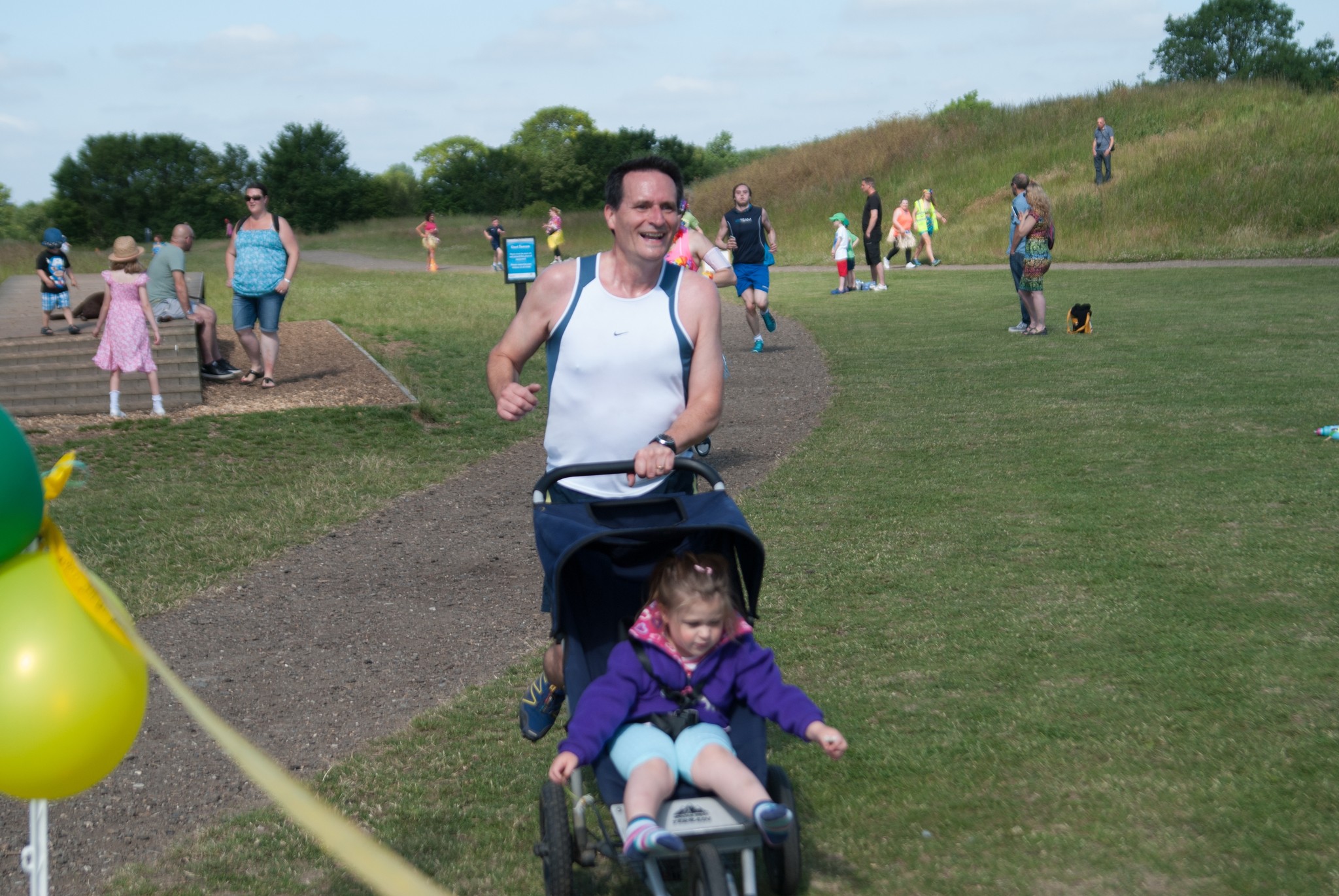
[0,549,149,799]
[0,405,43,566]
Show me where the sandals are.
[68,324,81,334]
[40,325,53,334]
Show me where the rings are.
[656,466,664,470]
[280,290,283,292]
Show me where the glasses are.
[245,194,261,201]
[185,235,196,239]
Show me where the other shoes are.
[491,264,504,271]
[1009,320,1027,331]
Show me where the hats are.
[107,235,144,262]
[40,227,67,247]
[829,213,845,221]
[841,218,850,227]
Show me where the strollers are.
[531,458,803,895]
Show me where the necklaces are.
[250,215,265,229]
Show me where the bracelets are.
[1109,147,1110,150]
[283,277,290,283]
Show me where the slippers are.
[830,287,849,294]
[261,377,275,389]
[1021,325,1047,335]
[238,369,264,385]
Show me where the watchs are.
[648,434,677,454]
[185,309,193,315]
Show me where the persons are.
[829,178,947,294]
[487,157,723,734]
[1006,173,1031,332]
[1019,181,1051,335]
[663,183,776,454]
[35,183,299,415]
[548,553,849,859]
[1092,117,1115,185]
[415,206,561,273]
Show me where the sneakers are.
[216,357,242,374]
[911,258,922,266]
[760,307,776,333]
[905,262,916,269]
[518,670,566,742]
[883,257,890,268]
[931,259,941,266]
[751,339,764,353]
[199,360,233,379]
[869,283,887,291]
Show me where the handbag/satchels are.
[1066,303,1093,334]
[547,229,564,248]
